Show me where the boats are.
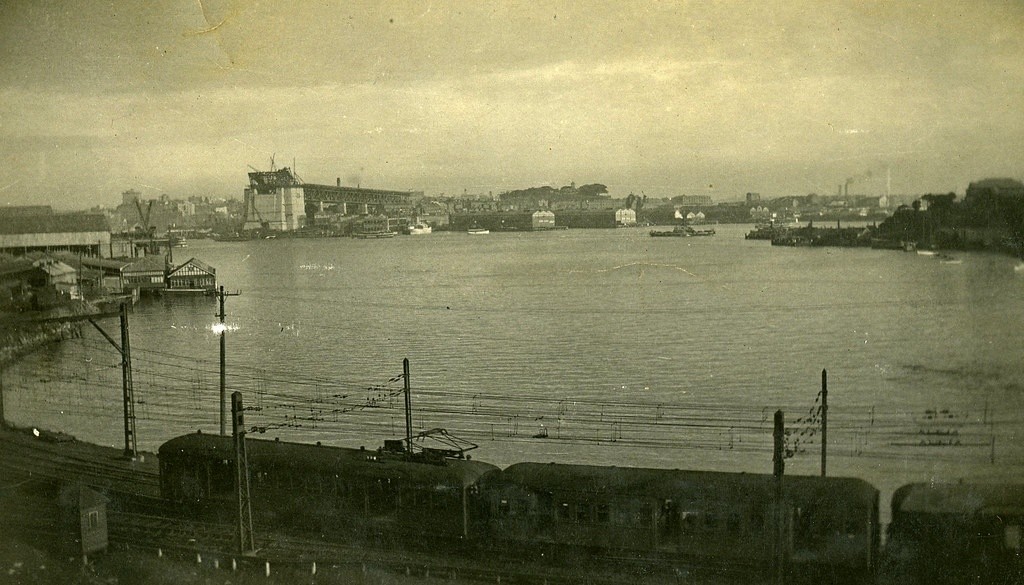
[649,220,717,236]
[402,221,432,235]
[358,231,393,239]
[467,229,489,235]
[745,221,918,249]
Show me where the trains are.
[155,433,1024,585]
